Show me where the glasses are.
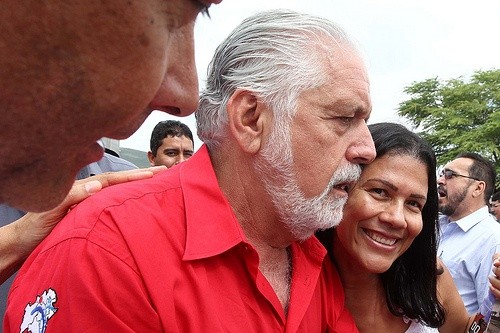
[440,171,481,182]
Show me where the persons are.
[490,192,500,221]
[0,0,223,285]
[147,120,194,167]
[0,151,140,317]
[2,10,376,333]
[316,123,500,333]
[435,152,500,333]
